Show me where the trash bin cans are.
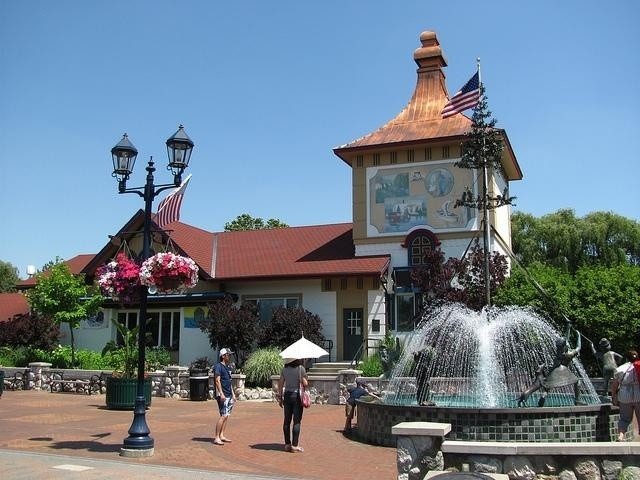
[188,368,209,401]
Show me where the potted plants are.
[188,356,215,378]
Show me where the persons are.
[534,329,585,408]
[612,349,639,441]
[340,379,384,432]
[212,347,236,447]
[277,357,308,453]
[590,338,625,400]
[378,338,400,391]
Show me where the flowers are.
[138,250,202,298]
[92,248,146,308]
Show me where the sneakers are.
[285,444,305,453]
[213,439,224,445]
[221,437,232,442]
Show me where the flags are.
[156,175,193,230]
[440,71,485,118]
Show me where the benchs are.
[1,365,31,392]
[49,371,113,396]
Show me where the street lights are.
[108,125,195,456]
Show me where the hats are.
[284,358,297,364]
[356,378,368,387]
[219,348,235,358]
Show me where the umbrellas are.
[279,335,330,370]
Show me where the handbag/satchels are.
[612,389,619,407]
[299,383,311,408]
